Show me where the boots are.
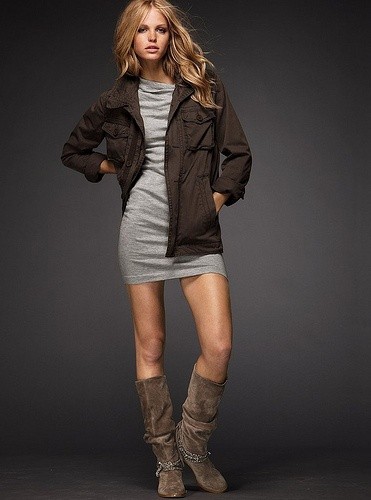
[132,372,187,496]
[176,360,229,494]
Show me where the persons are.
[61,1,254,500]
